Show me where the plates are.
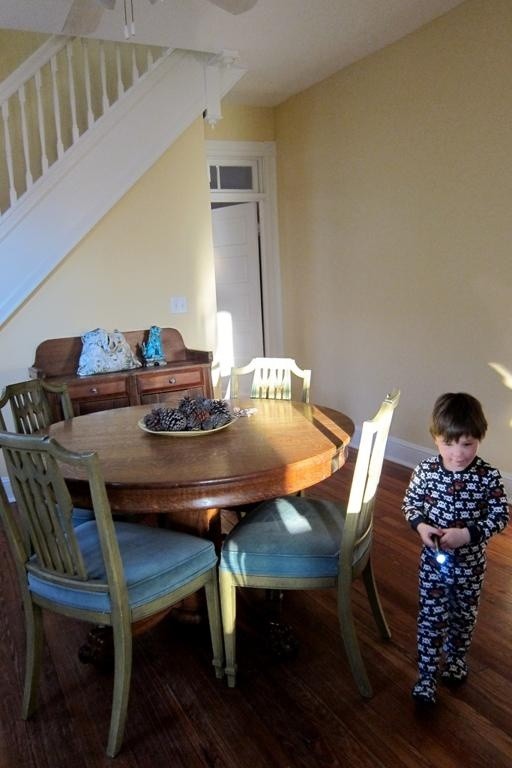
[137,406,239,438]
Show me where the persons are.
[403,391,511,708]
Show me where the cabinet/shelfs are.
[29,329,213,419]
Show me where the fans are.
[61,0,259,35]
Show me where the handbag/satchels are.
[77,328,142,376]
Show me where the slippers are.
[411,651,470,705]
